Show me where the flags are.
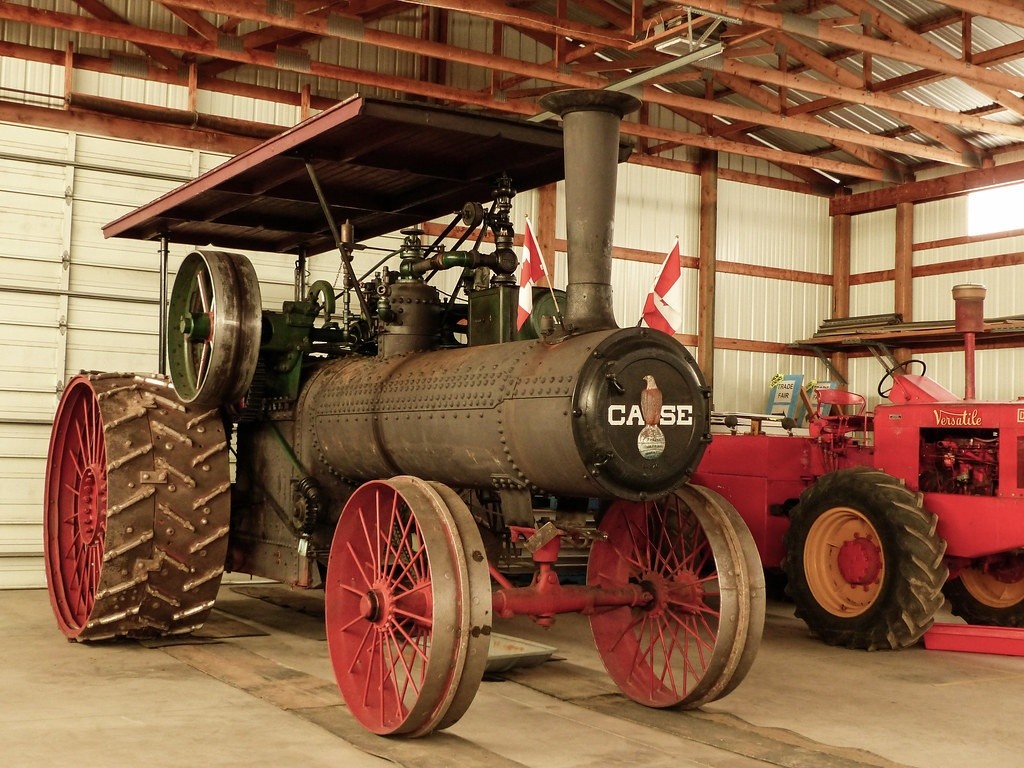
[517,218,548,334]
[644,239,682,339]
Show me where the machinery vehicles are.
[44,91,768,740]
[608,282,1024,656]
